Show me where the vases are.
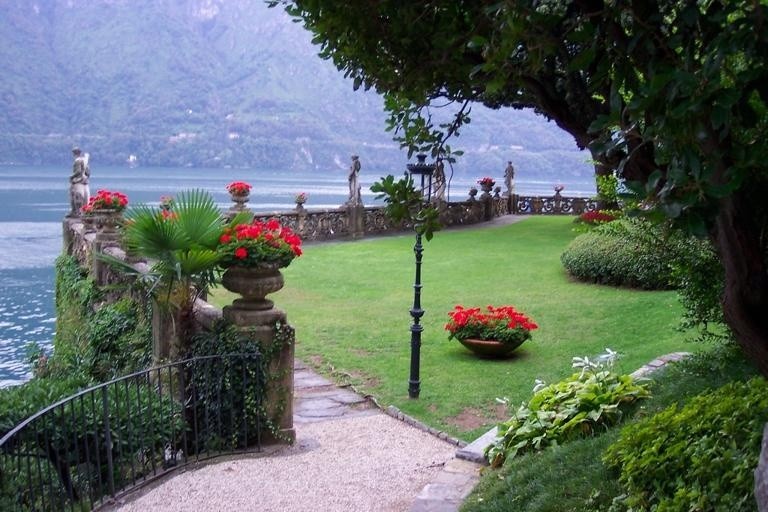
[229,193,250,207]
[456,338,527,362]
[296,201,304,208]
[479,184,493,191]
[81,216,94,229]
[160,205,171,212]
[89,208,125,231]
[219,262,287,310]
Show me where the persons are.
[501,159,513,193]
[346,156,362,205]
[66,147,90,217]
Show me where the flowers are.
[476,177,498,186]
[79,204,93,216]
[215,220,302,268]
[444,305,540,345]
[224,181,253,194]
[88,190,129,209]
[296,191,305,202]
[159,194,174,204]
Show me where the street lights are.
[405,152,439,398]
